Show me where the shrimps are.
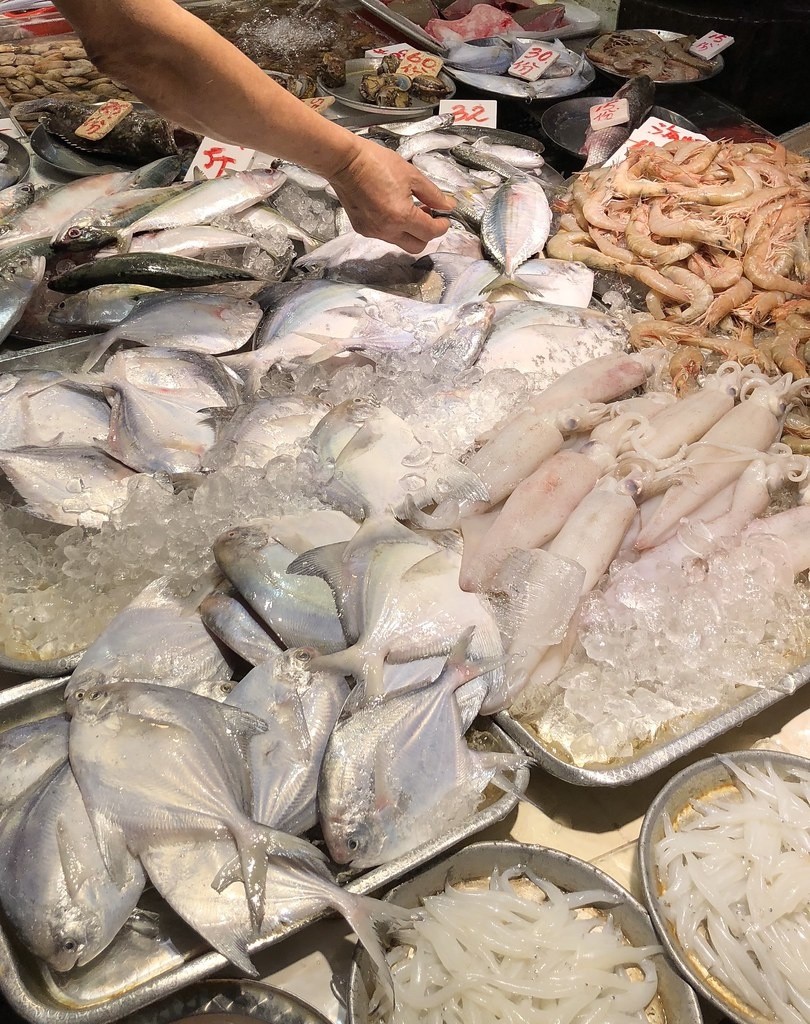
[548,136,810,455]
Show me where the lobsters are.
[585,29,715,81]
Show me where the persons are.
[51,0,456,255]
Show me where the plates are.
[316,58,457,115]
[30,100,202,174]
[262,69,305,99]
[582,28,726,84]
[637,749,810,1024]
[0,132,31,193]
[542,96,701,160]
[106,977,334,1024]
[348,841,705,1024]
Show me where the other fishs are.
[0,74,810,1024]
